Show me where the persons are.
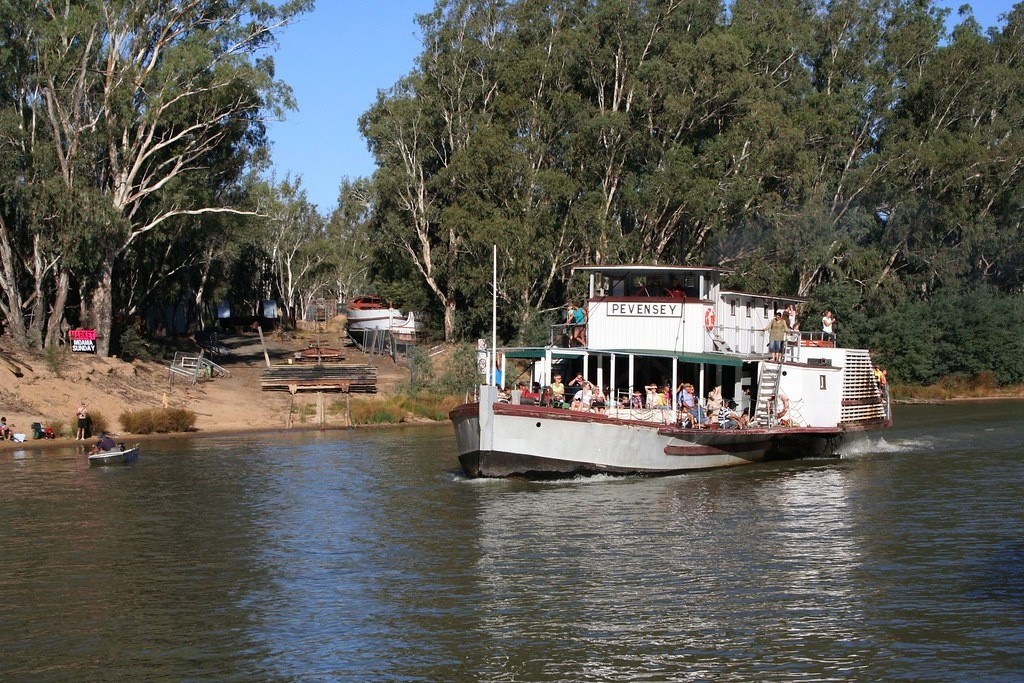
[562,301,588,347]
[763,312,790,364]
[496,373,759,431]
[783,303,800,346]
[1,417,13,441]
[822,309,836,342]
[76,403,88,441]
[89,431,117,456]
[873,365,887,397]
[666,278,688,298]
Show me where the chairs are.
[168,348,204,385]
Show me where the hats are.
[728,401,739,408]
[519,382,525,386]
[875,365,879,367]
[98,432,106,438]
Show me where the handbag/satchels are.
[31,422,45,439]
[592,402,605,409]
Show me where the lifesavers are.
[705,307,716,332]
[767,394,789,419]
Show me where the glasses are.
[687,386,691,387]
[556,378,561,380]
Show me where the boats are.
[88,442,140,467]
[448,244,893,479]
[345,294,424,348]
